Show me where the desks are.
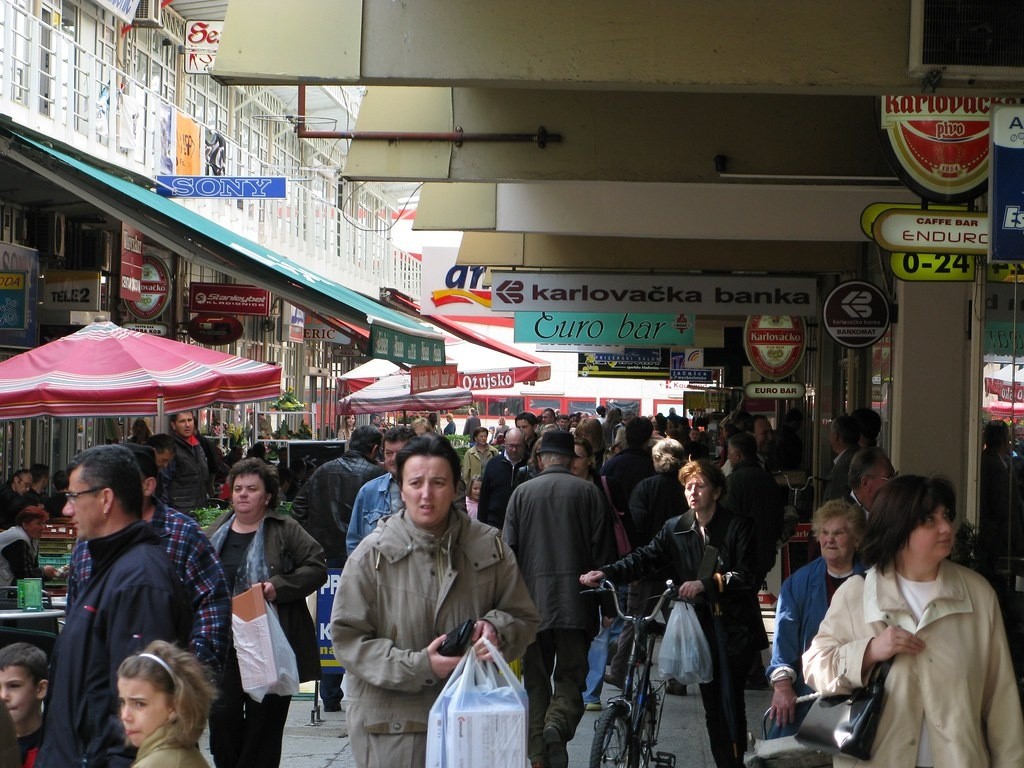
[0,609,65,635]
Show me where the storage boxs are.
[36,519,77,597]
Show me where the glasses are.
[56,486,119,504]
[18,477,32,488]
[505,442,522,450]
[866,471,899,483]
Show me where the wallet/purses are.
[438,619,476,656]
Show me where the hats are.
[536,431,582,458]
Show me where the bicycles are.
[580,578,707,768]
[772,470,829,509]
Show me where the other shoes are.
[602,672,624,689]
[744,661,768,690]
[666,685,687,696]
[324,702,341,712]
[534,725,568,767]
[584,703,601,711]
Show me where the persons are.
[0,404,1024,768]
[976,414,1024,652]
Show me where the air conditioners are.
[133,0,164,28]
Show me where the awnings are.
[0,118,445,367]
[379,284,553,383]
[266,286,461,393]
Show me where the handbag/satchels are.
[601,475,631,559]
[425,638,532,768]
[658,597,713,684]
[231,596,300,702]
[714,573,766,655]
[794,572,895,760]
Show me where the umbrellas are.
[983,361,1024,419]
[335,373,474,427]
[335,328,540,396]
[0,313,282,445]
